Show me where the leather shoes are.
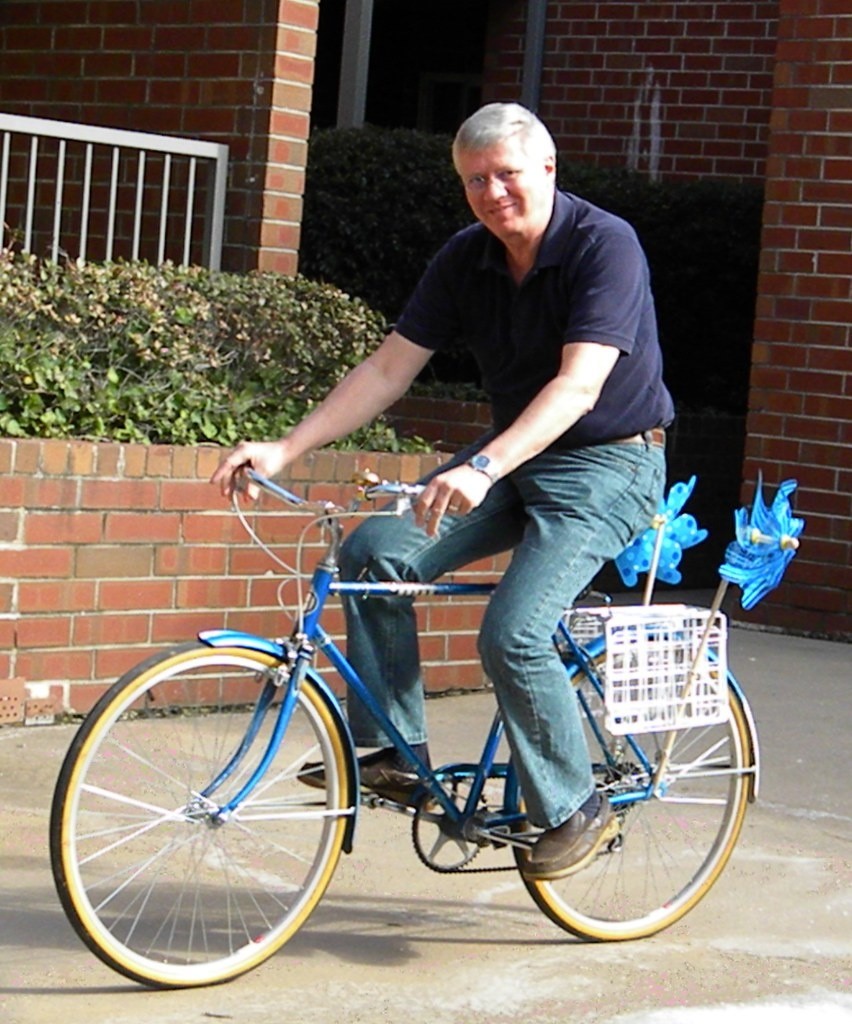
[522,791,620,880]
[297,750,437,811]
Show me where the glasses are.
[465,158,545,193]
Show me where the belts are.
[611,429,665,447]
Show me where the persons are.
[209,102,674,883]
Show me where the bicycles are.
[47,465,760,991]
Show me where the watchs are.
[465,454,498,486]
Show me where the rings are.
[448,505,458,512]
